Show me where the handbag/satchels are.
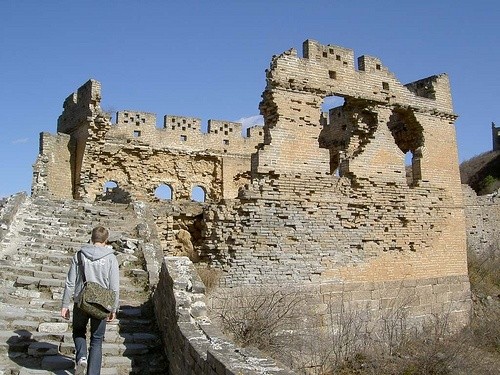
[77,281,116,320]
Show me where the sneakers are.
[73,356,87,375]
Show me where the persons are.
[60,225,120,375]
